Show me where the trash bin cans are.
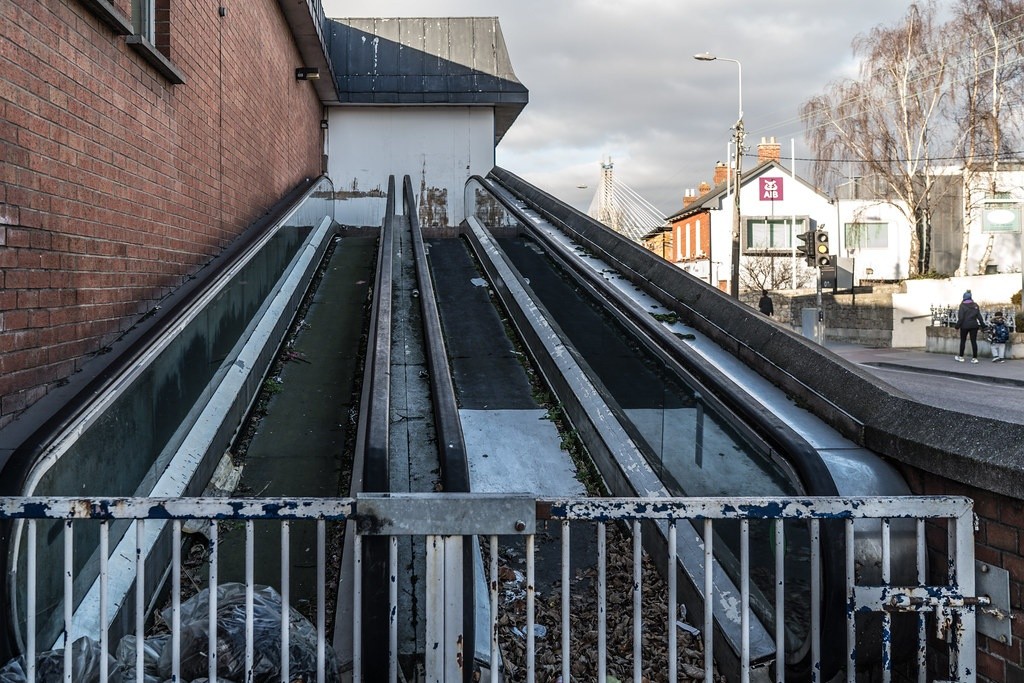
[801,307,818,342]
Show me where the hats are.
[963,289,972,300]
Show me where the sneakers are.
[955,355,964,362]
[992,356,1005,363]
[971,358,979,364]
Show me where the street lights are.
[693,54,743,299]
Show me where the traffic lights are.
[796,231,815,267]
[815,232,830,267]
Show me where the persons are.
[954,290,987,364]
[759,290,774,316]
[985,312,1009,363]
